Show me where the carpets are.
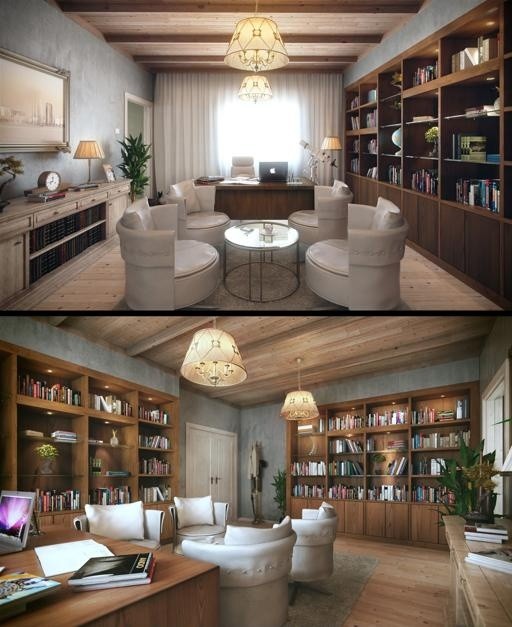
[290,553,378,627]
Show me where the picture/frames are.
[0,46,70,154]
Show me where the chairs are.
[229,154,256,180]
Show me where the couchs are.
[286,177,355,263]
[304,194,409,312]
[158,179,232,248]
[115,194,222,310]
[166,501,229,553]
[270,507,340,605]
[177,521,298,625]
[71,505,165,549]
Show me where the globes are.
[392,128,401,155]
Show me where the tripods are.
[310,159,320,185]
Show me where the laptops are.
[0,491,36,555]
[257,162,288,183]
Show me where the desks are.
[196,175,319,223]
[1,521,224,627]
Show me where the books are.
[345,34,500,215]
[460,517,510,575]
[18,357,174,516]
[0,537,157,624]
[69,181,101,192]
[194,175,260,186]
[286,397,472,506]
[27,201,107,286]
[25,191,66,203]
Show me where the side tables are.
[222,220,300,303]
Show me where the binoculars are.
[299,139,338,186]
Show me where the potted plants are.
[422,124,439,158]
[32,443,61,474]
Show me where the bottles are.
[110,428,119,445]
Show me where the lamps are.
[277,355,321,422]
[238,71,273,105]
[72,139,107,190]
[320,134,342,188]
[220,0,291,73]
[176,315,249,388]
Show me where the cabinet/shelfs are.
[283,378,479,546]
[343,1,510,311]
[0,175,133,303]
[0,340,182,543]
[439,510,511,626]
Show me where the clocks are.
[36,172,61,192]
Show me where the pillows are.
[329,179,351,196]
[122,196,154,233]
[224,513,293,548]
[83,497,147,541]
[370,195,403,232]
[169,179,203,214]
[314,500,337,519]
[171,492,215,529]
